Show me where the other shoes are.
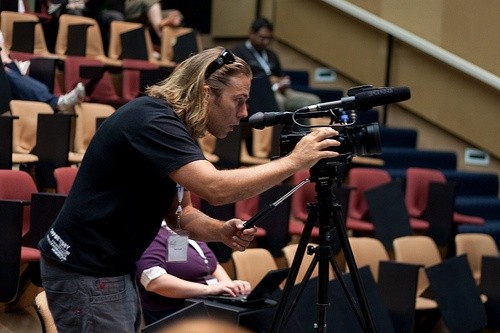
[57,82,85,113]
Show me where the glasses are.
[204,48,236,80]
[256,33,275,41]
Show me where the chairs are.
[0,10,500,333]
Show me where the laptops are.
[207,267,291,305]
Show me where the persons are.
[155,315,251,333]
[37,46,340,333]
[231,16,321,113]
[0,31,85,114]
[135,219,252,325]
[17,0,203,57]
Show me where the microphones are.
[342,86,411,109]
[248,111,335,130]
